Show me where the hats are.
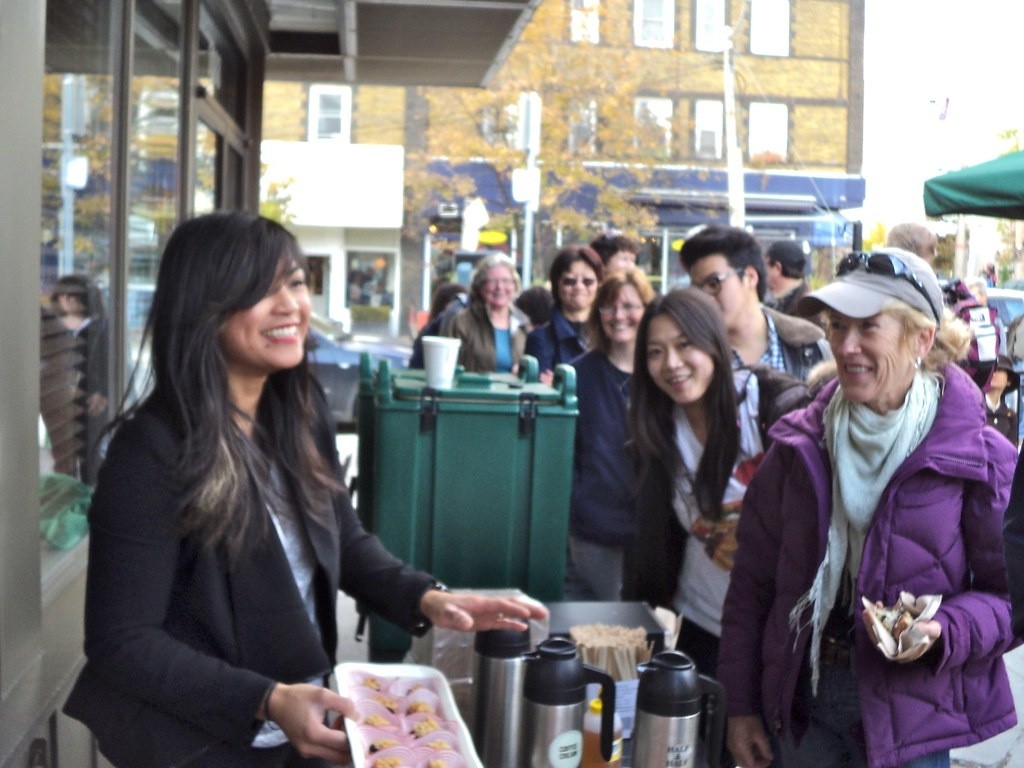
[797,247,945,321]
[770,237,811,278]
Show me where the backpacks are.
[945,280,1001,393]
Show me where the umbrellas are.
[921,153,1023,225]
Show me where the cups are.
[421,334,464,390]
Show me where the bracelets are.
[413,580,448,623]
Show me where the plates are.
[333,660,483,767]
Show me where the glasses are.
[560,275,600,286]
[837,252,939,320]
[688,269,743,295]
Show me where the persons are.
[713,248,1018,767]
[405,217,1024,679]
[60,212,548,767]
[37,274,125,487]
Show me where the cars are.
[300,307,413,432]
[984,284,1024,373]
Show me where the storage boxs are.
[358,349,580,663]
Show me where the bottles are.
[469,608,725,768]
